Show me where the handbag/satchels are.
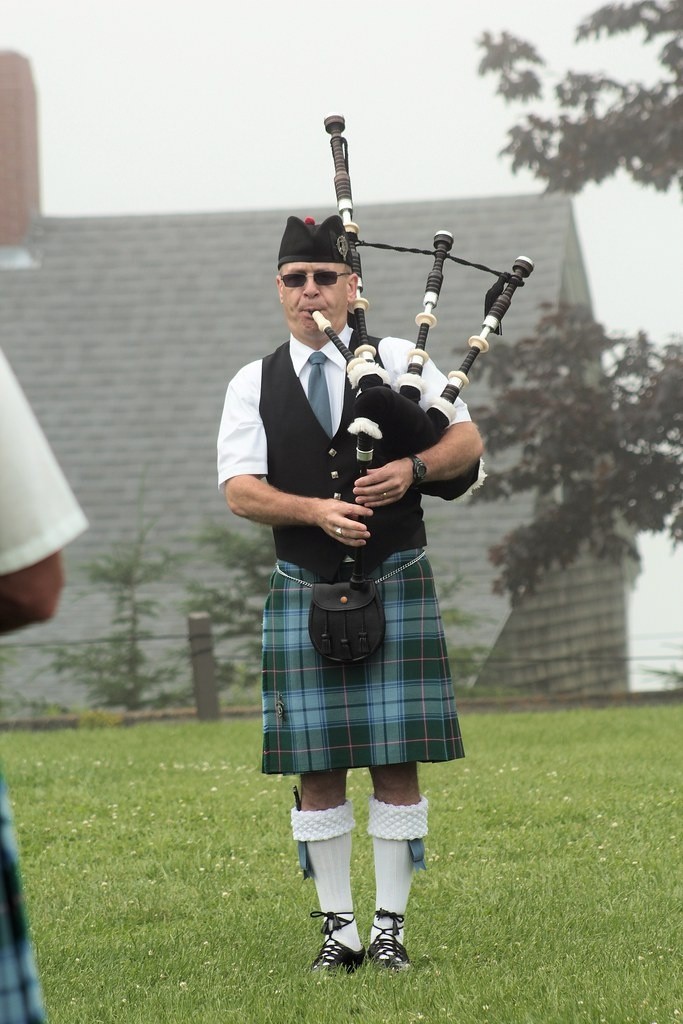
[308,580,386,663]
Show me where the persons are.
[0,342,89,1024]
[216,215,484,977]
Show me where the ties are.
[308,352,332,437]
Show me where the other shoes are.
[366,908,409,969]
[311,910,365,973]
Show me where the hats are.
[278,215,352,269]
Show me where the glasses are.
[281,271,352,287]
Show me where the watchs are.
[408,454,427,484]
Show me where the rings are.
[384,492,387,499]
[335,528,342,536]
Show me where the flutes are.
[311,113,539,592]
